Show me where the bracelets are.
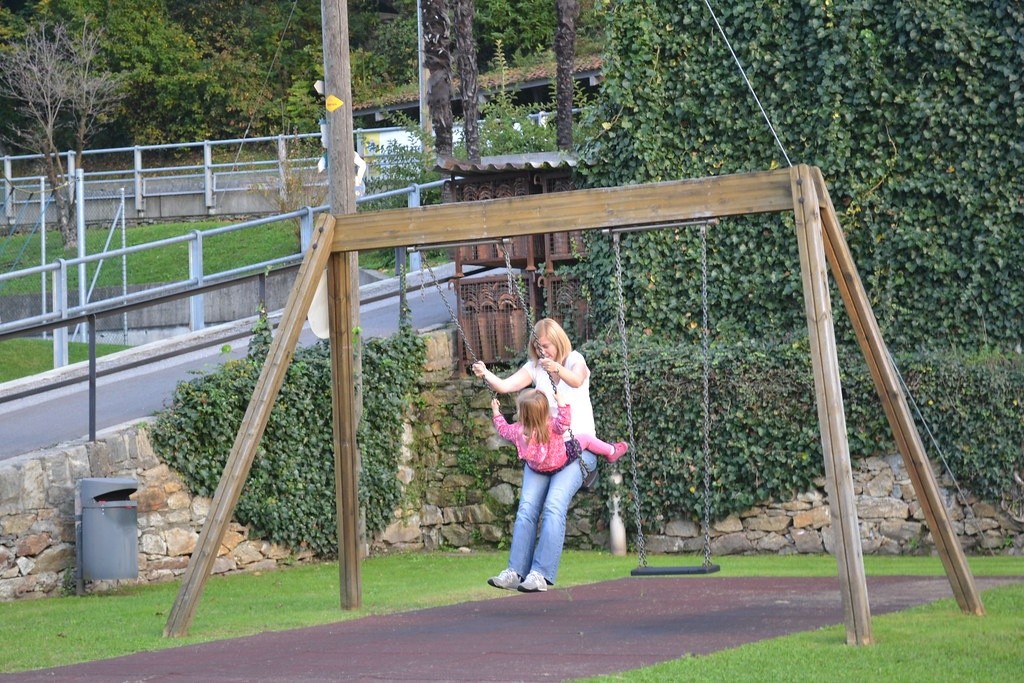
[555,362,561,374]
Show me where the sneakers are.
[487,568,521,589]
[517,569,548,592]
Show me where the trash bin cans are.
[78,477,139,581]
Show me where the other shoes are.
[605,441,628,463]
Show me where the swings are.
[612,227,723,575]
[418,243,600,488]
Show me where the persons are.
[471,317,598,594]
[489,389,629,475]
[315,118,367,206]
